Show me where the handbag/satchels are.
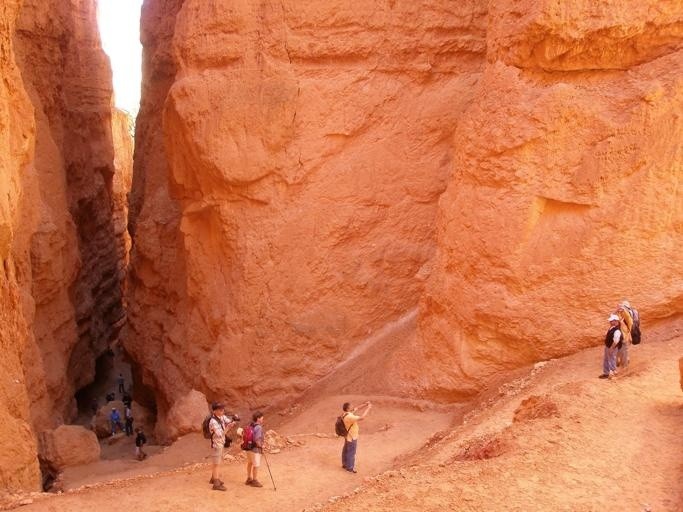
[224,434,233,448]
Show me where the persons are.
[208,401,237,491]
[341,400,372,474]
[597,300,634,380]
[91,373,147,462]
[244,411,266,488]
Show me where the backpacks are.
[631,323,641,345]
[632,309,640,327]
[334,413,353,437]
[617,328,624,350]
[241,423,259,449]
[202,414,224,440]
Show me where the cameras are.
[232,415,240,421]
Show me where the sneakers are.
[209,478,224,484]
[598,373,609,379]
[342,465,357,473]
[245,477,252,485]
[250,481,264,487]
[212,484,227,490]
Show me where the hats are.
[212,401,227,411]
[607,314,620,321]
[618,300,633,317]
